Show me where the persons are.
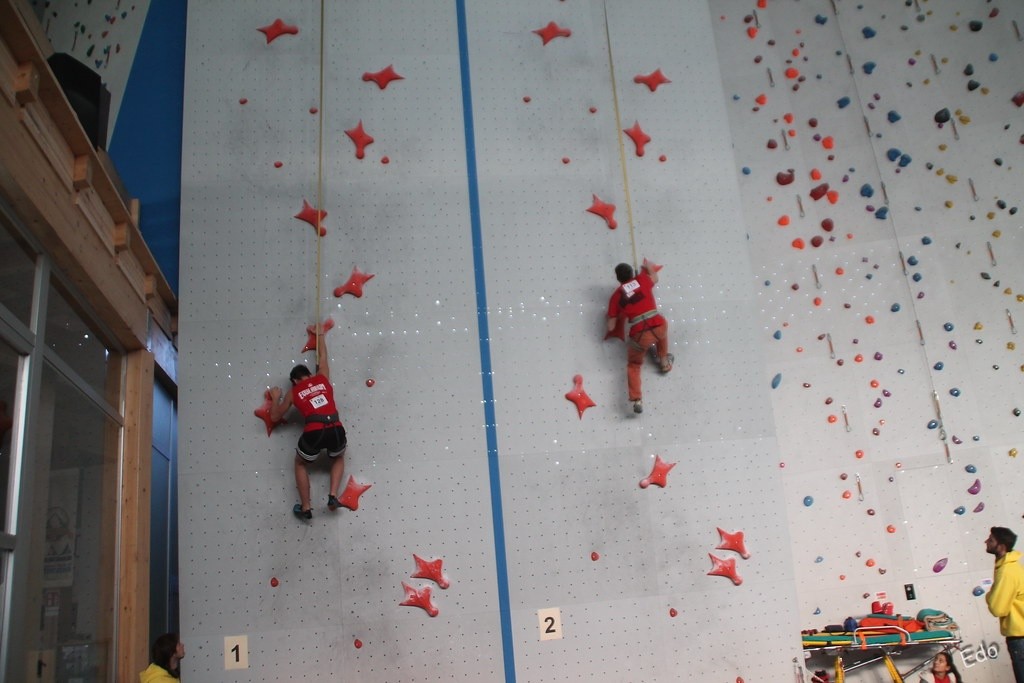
[267,322,347,519]
[139,633,185,683]
[919,652,961,683]
[607,258,674,414]
[984,526,1024,683]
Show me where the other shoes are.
[328,494,340,511]
[634,400,642,413]
[293,504,313,519]
[660,352,674,372]
